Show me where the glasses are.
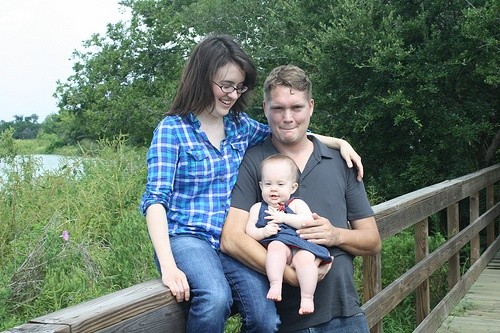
[211,79,248,94]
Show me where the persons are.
[246,153,332,316]
[139,34,363,333]
[218,64,382,333]
